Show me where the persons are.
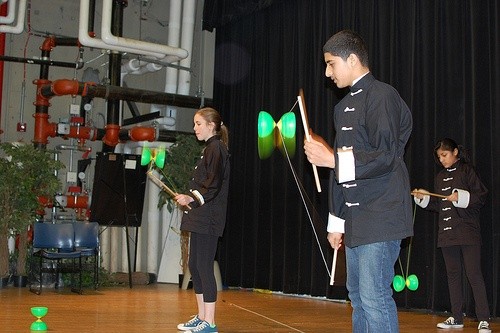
[173,108,231,333]
[303,29,414,333]
[411,138,492,333]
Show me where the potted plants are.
[0,141,66,288]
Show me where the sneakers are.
[177,314,203,330]
[437,317,464,328]
[478,320,491,333]
[184,320,218,333]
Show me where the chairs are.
[28,222,82,295]
[55,222,100,293]
